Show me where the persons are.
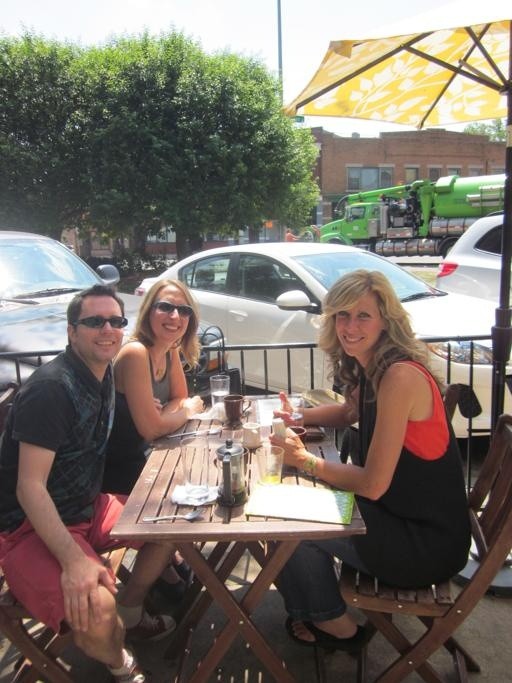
[258,263,472,656]
[1,283,182,681]
[284,226,302,241]
[105,278,210,616]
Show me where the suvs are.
[435,207,511,308]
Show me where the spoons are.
[166,425,224,439]
[142,507,204,522]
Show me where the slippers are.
[284,613,368,648]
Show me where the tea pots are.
[215,438,248,506]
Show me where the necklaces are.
[151,347,169,381]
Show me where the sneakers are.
[122,613,177,645]
[107,643,152,683]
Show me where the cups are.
[224,394,252,425]
[213,447,248,485]
[255,445,286,487]
[242,421,261,449]
[289,425,307,443]
[281,395,305,427]
[210,373,231,406]
[180,436,210,500]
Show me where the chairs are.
[337,411,511,683]
[0,543,169,682]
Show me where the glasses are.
[152,298,195,318]
[75,315,129,328]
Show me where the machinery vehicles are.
[306,171,506,268]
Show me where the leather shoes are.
[153,558,194,589]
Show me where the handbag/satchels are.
[186,323,242,398]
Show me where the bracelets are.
[301,452,320,480]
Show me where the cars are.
[0,226,230,400]
[134,241,511,437]
